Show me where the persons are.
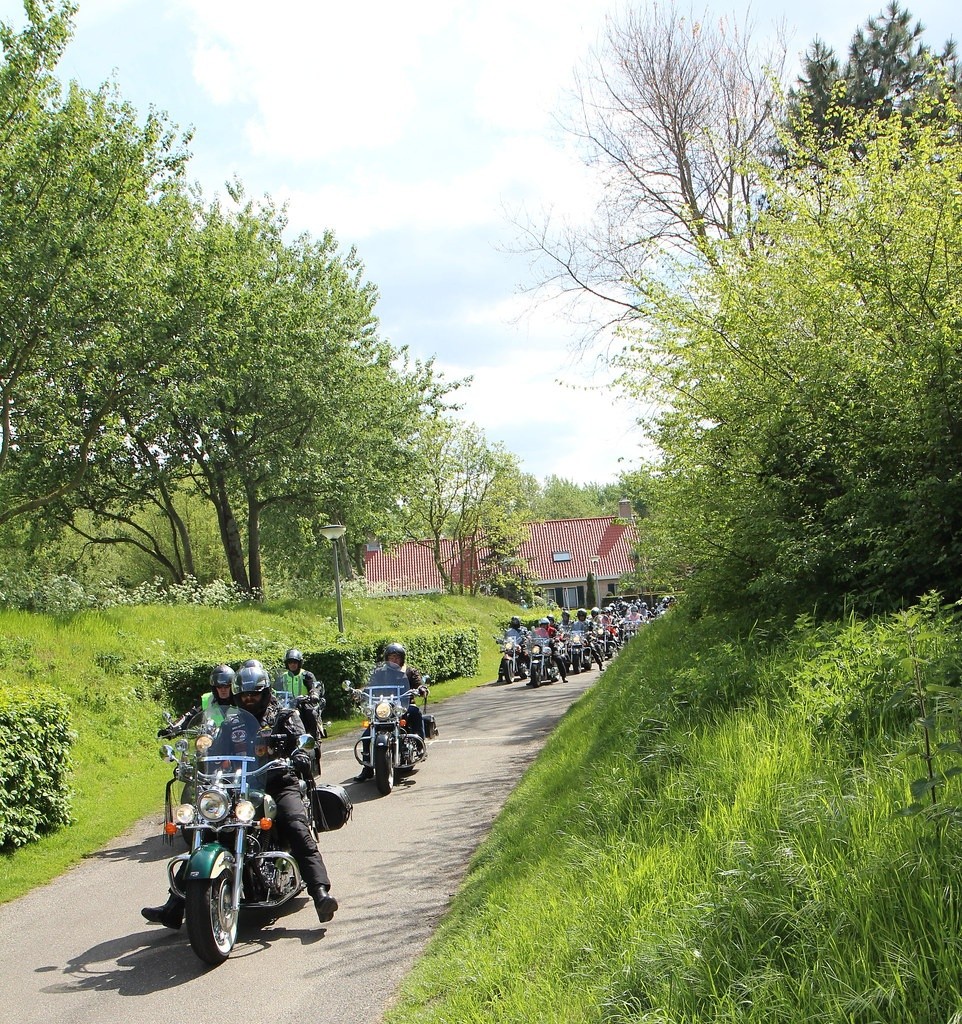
[141,667,339,931]
[525,618,570,685]
[276,647,322,745]
[587,595,671,662]
[496,616,528,682]
[546,614,564,636]
[158,665,238,740]
[243,659,281,699]
[571,608,606,672]
[558,610,574,632]
[352,643,429,782]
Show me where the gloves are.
[310,693,319,702]
[294,754,313,772]
[157,728,173,742]
[419,686,428,698]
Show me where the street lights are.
[319,523,347,631]
[589,554,601,612]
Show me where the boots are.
[314,885,338,923]
[142,888,185,929]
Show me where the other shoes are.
[600,666,605,671]
[563,679,568,683]
[497,678,503,683]
[417,749,427,758]
[526,680,532,685]
[353,767,373,783]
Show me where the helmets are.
[241,660,264,672]
[384,644,407,663]
[511,596,675,628]
[232,668,271,696]
[209,666,235,687]
[285,648,302,663]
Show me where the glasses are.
[238,691,261,697]
[216,684,230,689]
[287,659,298,664]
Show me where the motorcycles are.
[525,628,563,687]
[273,691,332,776]
[152,703,309,967]
[341,659,432,797]
[494,628,531,684]
[557,612,645,678]
